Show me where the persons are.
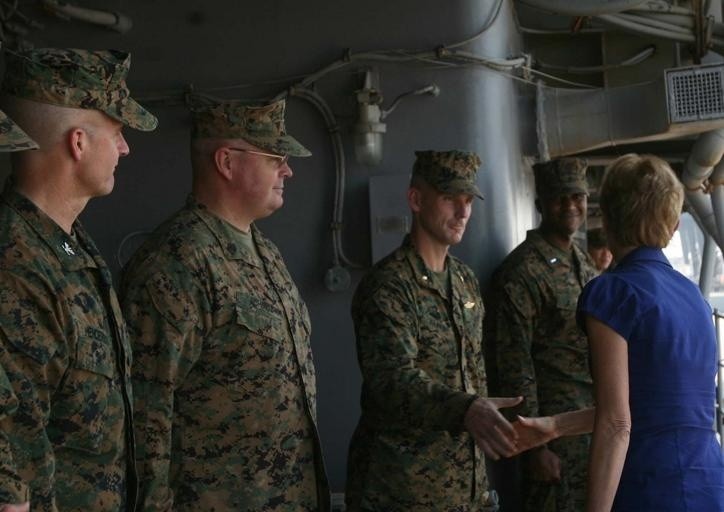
[0,0,159,512]
[588,227,614,275]
[483,157,607,512]
[343,150,524,512]
[118,88,333,512]
[504,151,724,512]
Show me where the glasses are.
[228,146,289,168]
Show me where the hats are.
[530,153,590,201]
[0,39,160,133]
[585,227,611,250]
[412,149,485,202]
[0,108,42,155]
[186,87,313,158]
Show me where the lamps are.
[344,78,440,176]
